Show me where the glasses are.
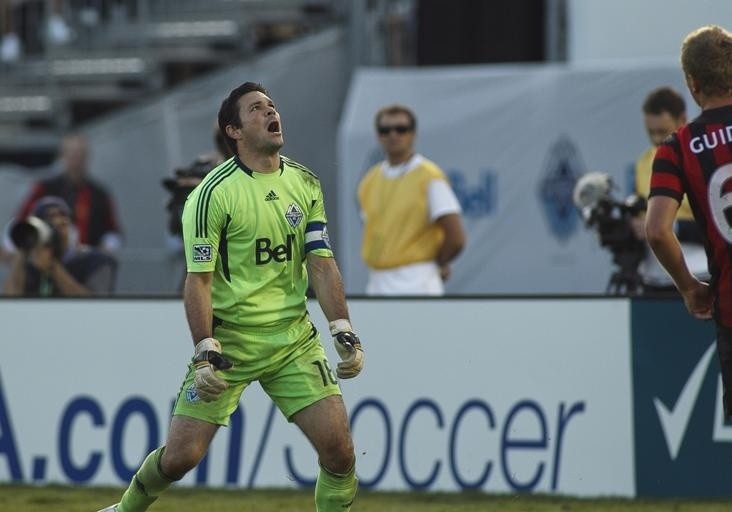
[378,126,410,134]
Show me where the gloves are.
[192,338,235,403]
[328,319,364,379]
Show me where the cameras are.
[8,216,62,256]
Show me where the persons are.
[87,81,365,512]
[4,195,119,298]
[158,119,235,293]
[643,23,732,433]
[626,82,714,299]
[352,103,468,298]
[14,133,130,262]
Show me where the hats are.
[572,172,613,227]
